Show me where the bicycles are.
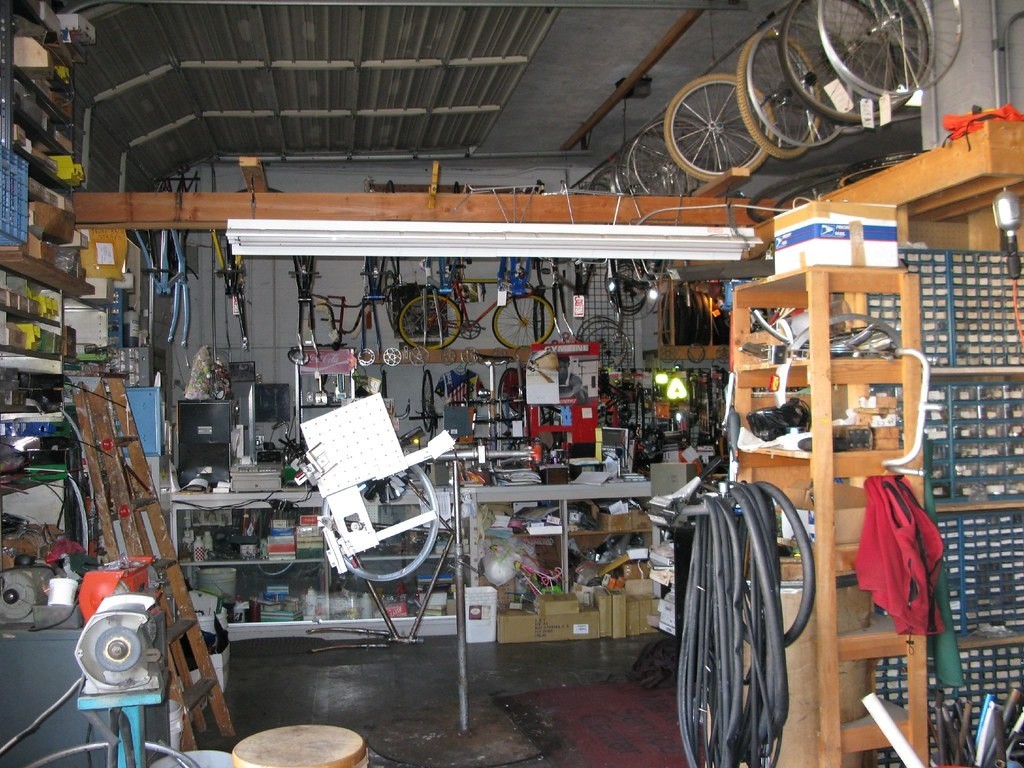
[398,264,557,352]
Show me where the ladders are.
[63,370,236,752]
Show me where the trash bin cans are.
[453,586,497,643]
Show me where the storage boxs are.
[185,504,676,693]
[0,13,89,467]
[774,200,898,273]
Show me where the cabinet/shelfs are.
[732,263,930,768]
[168,483,651,645]
[0,0,95,565]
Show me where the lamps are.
[224,220,764,264]
